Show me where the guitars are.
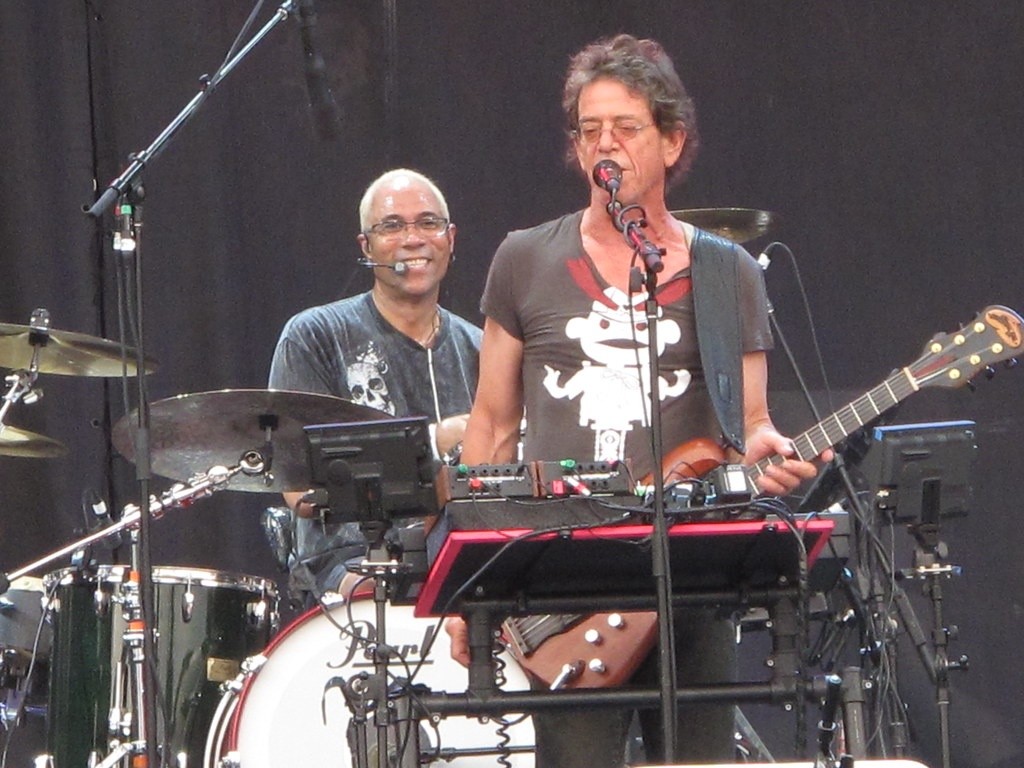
[500,303,1024,692]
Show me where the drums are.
[0,562,282,768]
[203,587,537,768]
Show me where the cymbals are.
[0,420,70,459]
[111,387,398,495]
[669,207,781,247]
[0,320,161,380]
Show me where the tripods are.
[0,425,274,768]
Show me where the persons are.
[262,168,488,607]
[457,35,838,768]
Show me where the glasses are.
[365,217,450,240]
[572,120,658,144]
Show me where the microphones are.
[295,0,342,143]
[757,244,776,271]
[357,257,409,275]
[593,160,623,192]
[90,491,122,549]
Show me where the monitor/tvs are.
[303,416,441,524]
[872,420,976,527]
